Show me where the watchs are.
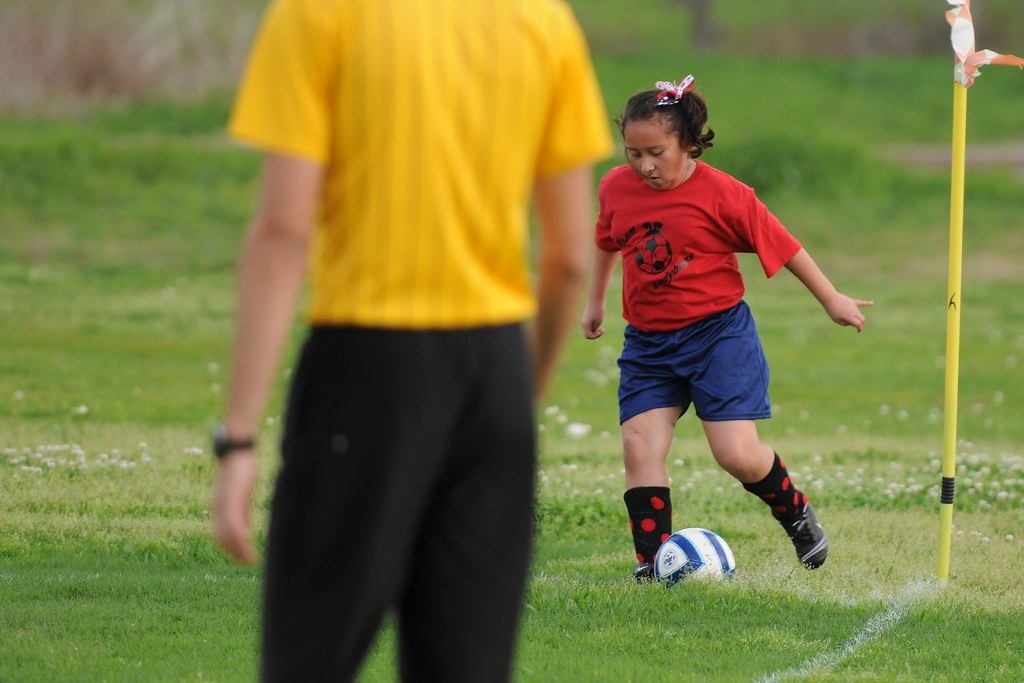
[211,427,254,458]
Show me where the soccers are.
[654,528,736,590]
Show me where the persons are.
[581,75,874,583]
[213,0,612,683]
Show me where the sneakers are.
[631,562,653,583]
[778,504,829,570]
